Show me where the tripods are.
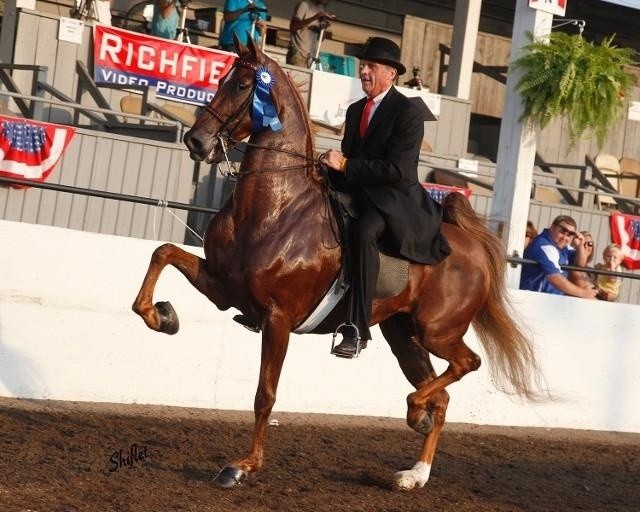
[174,7,191,45]
[309,29,324,72]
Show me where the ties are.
[359,97,375,141]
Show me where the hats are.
[356,37,406,75]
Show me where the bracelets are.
[339,155,348,174]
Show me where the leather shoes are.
[333,336,367,355]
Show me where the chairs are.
[594,153,640,214]
[121,97,197,129]
[467,176,562,205]
[318,52,357,78]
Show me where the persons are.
[317,36,452,360]
[518,215,624,302]
[219,0,268,54]
[149,0,188,42]
[286,0,337,70]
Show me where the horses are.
[131,28,563,490]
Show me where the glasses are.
[585,241,593,246]
[556,223,577,236]
